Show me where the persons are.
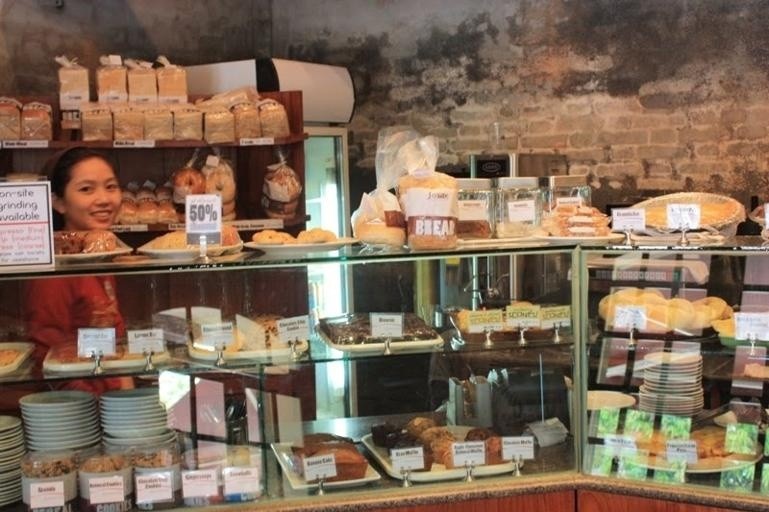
[25,146,133,430]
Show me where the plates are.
[311,321,446,353]
[50,246,136,264]
[135,241,242,259]
[243,235,360,254]
[639,351,705,419]
[607,428,764,474]
[271,441,382,491]
[184,332,310,361]
[457,236,546,246]
[0,389,180,512]
[0,339,38,375]
[42,340,172,373]
[544,233,625,245]
[359,432,530,483]
[583,389,637,412]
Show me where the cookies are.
[253,227,337,243]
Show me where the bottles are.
[540,173,592,229]
[494,175,543,238]
[456,176,497,241]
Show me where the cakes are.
[229,311,296,350]
[117,340,145,359]
[292,432,368,481]
[55,230,120,255]
[457,300,555,341]
[372,417,512,473]
[58,340,92,362]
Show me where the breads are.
[598,287,737,337]
[634,424,733,459]
[79,53,289,143]
[355,212,406,246]
[55,54,90,130]
[743,362,769,378]
[398,174,459,250]
[0,96,55,141]
[151,225,240,250]
[0,349,19,365]
[257,150,301,220]
[319,312,437,345]
[1,147,235,223]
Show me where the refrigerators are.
[170,57,362,433]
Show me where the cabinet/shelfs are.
[1,124,354,436]
[0,234,768,512]
